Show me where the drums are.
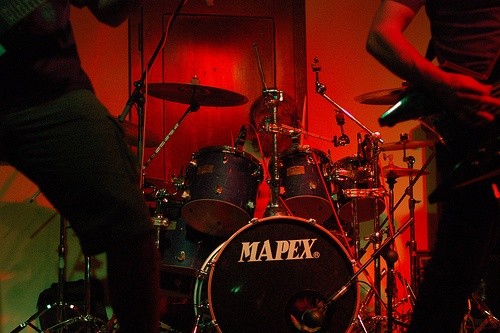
[266,145,334,222]
[332,155,386,223]
[151,191,215,273]
[180,144,264,236]
[194,214,361,333]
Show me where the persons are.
[365,0,500,333]
[244,93,301,218]
[0,0,160,333]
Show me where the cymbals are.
[145,82,251,107]
[354,88,409,104]
[381,164,431,177]
[109,115,168,147]
[378,139,436,151]
